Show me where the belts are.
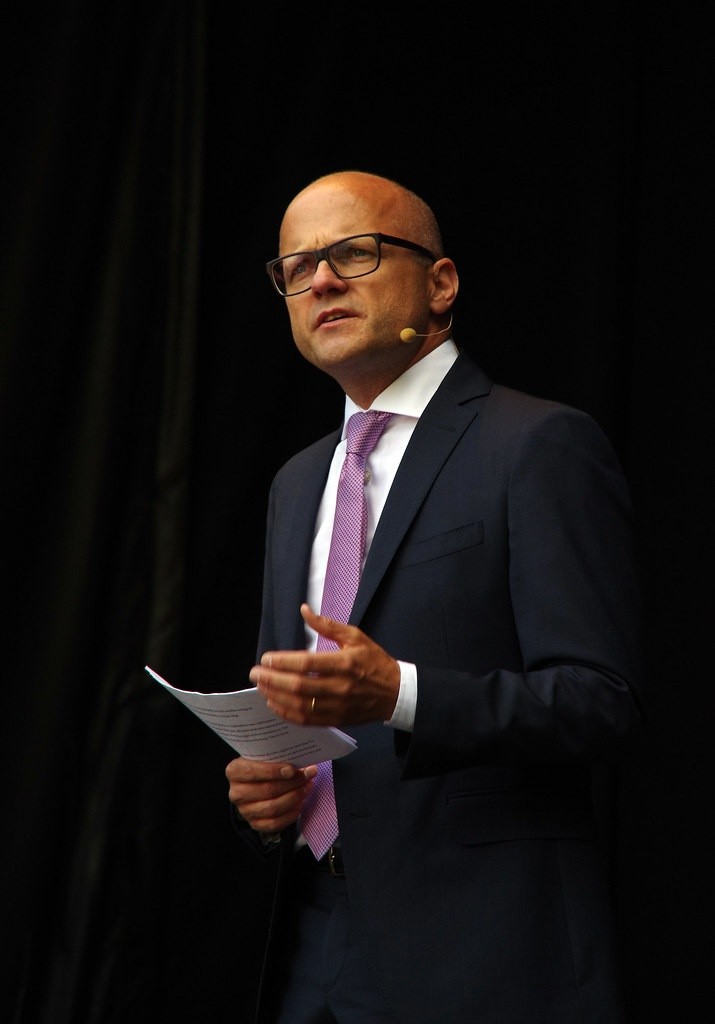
[297,846,344,878]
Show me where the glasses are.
[267,231,438,297]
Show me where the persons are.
[225,169,669,1024]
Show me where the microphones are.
[400,314,452,342]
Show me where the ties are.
[293,412,390,861]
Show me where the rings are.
[311,696,316,714]
